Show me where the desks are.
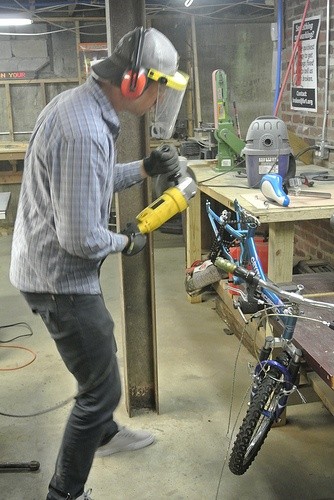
[183,159,334,429]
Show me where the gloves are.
[142,143,181,177]
[121,221,148,255]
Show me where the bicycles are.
[184,198,333,475]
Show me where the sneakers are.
[42,487,94,500]
[93,425,154,456]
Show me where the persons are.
[9,27,198,500]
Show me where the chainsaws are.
[122,147,199,246]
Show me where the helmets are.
[91,27,189,89]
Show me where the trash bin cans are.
[240,115,291,189]
